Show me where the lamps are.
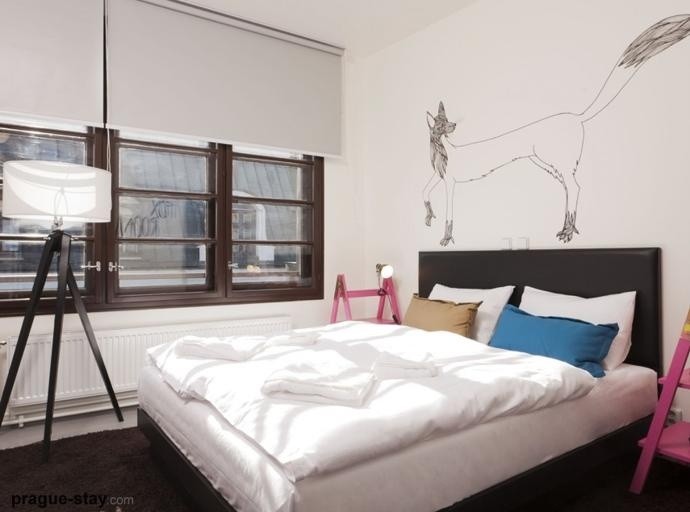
[0,160,125,462]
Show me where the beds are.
[136,246,662,512]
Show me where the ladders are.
[330,270,397,324]
[631,312,690,494]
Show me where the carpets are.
[1,425,687,512]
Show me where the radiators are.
[2,315,294,429]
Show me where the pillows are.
[403,282,638,377]
